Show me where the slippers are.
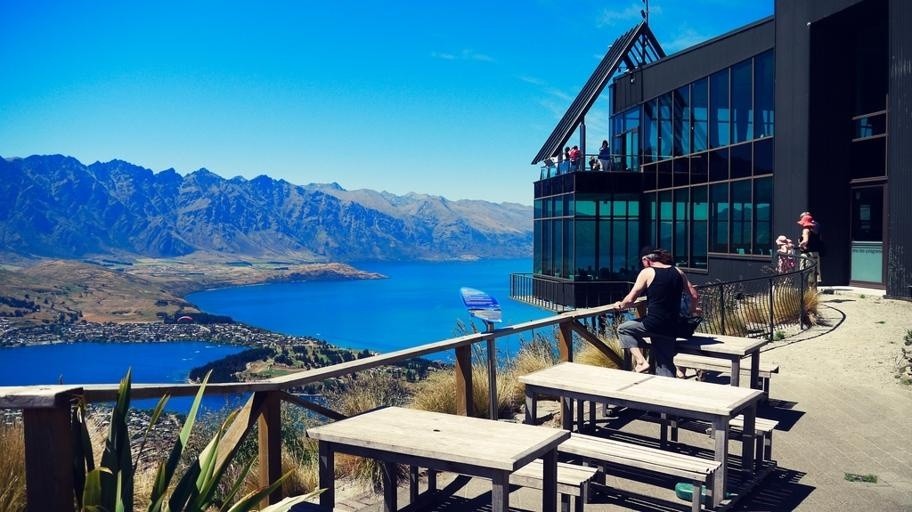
[632,368,649,373]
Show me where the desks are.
[306,403,573,512]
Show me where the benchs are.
[427,331,779,512]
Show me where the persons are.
[618,293,702,350]
[565,147,572,173]
[795,211,822,294]
[570,146,581,172]
[589,156,600,171]
[598,140,611,171]
[775,235,796,285]
[608,245,699,377]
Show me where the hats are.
[798,215,815,226]
[640,247,662,257]
[776,235,792,245]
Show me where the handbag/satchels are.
[676,292,702,338]
